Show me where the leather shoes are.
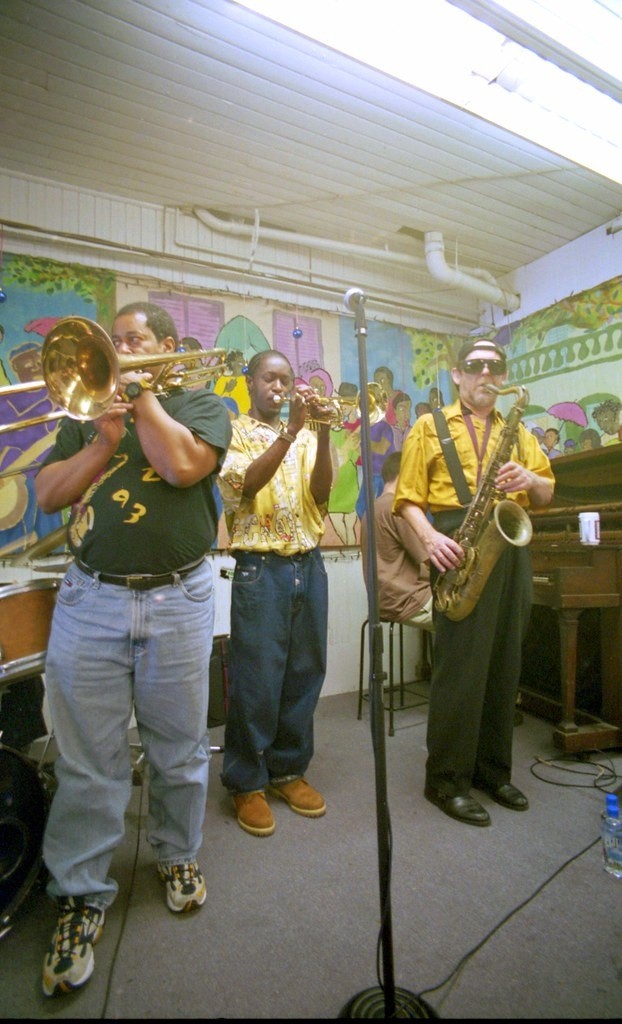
[423,786,491,826]
[474,781,529,810]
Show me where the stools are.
[358,618,434,737]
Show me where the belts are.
[73,556,206,589]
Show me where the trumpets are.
[272,383,389,432]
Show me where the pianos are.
[416,445,622,754]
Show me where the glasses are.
[458,359,509,375]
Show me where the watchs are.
[119,381,154,404]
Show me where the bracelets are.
[280,429,296,443]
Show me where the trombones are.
[0,315,231,432]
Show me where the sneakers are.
[158,862,207,913]
[232,792,275,837]
[268,777,326,817]
[42,907,105,996]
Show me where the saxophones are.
[433,383,533,622]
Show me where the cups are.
[578,512,601,545]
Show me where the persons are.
[216,349,335,836]
[361,452,523,728]
[34,301,232,997]
[393,338,556,826]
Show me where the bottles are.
[605,804,622,877]
[601,792,622,871]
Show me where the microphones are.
[344,287,366,310]
